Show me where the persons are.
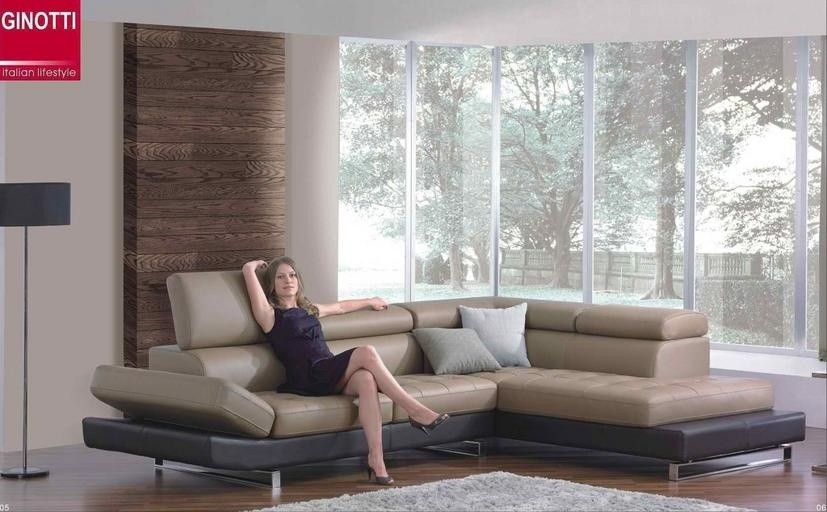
[242,255,449,485]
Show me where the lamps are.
[0,183,70,479]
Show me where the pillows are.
[411,327,502,375]
[456,302,533,368]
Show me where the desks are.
[811,372,827,473]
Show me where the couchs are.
[81,269,805,486]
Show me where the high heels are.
[409,414,450,436]
[368,463,394,485]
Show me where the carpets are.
[241,469,755,512]
